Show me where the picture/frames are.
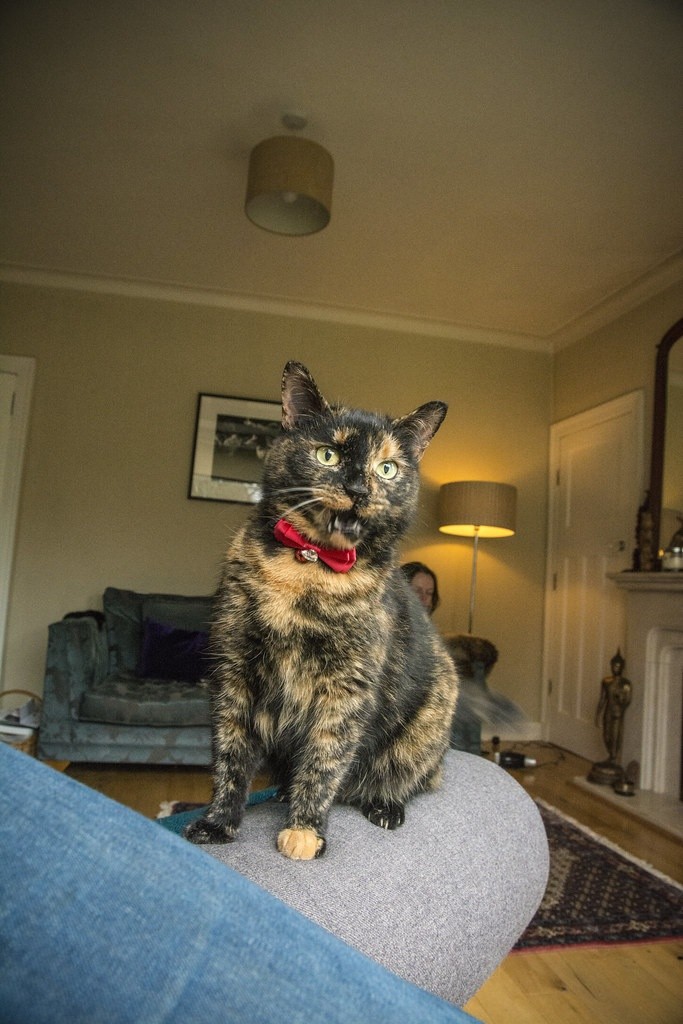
[186,392,282,504]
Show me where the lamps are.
[438,481,517,632]
[244,114,334,236]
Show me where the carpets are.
[507,796,682,952]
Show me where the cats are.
[185,359,460,861]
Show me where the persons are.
[593,646,632,764]
[397,556,440,620]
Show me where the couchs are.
[35,588,215,771]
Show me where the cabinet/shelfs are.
[612,574,682,799]
